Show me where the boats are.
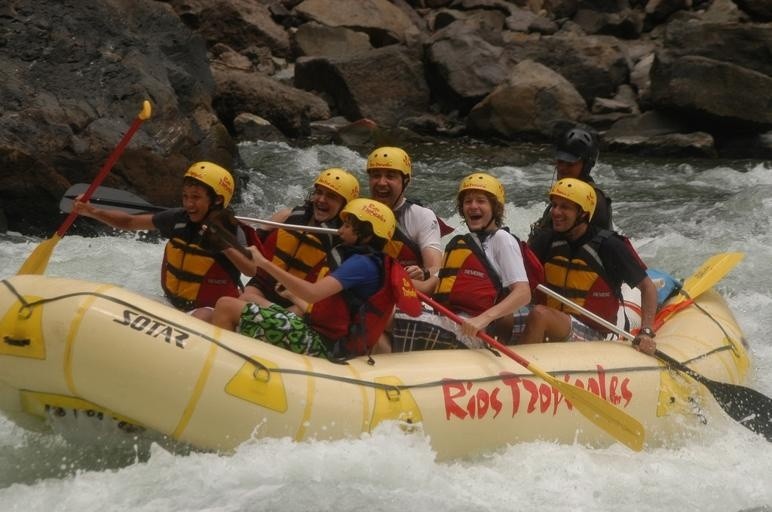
[0,267,752,463]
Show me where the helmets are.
[457,173,505,230]
[367,146,412,208]
[182,160,235,224]
[554,127,597,181]
[550,179,598,234]
[339,198,396,249]
[312,168,360,222]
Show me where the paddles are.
[16,100,151,274]
[59,184,340,237]
[416,291,644,453]
[537,283,772,442]
[640,252,742,335]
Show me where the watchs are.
[639,328,655,338]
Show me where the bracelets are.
[421,267,430,280]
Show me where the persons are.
[525,127,612,265]
[367,145,444,281]
[495,177,658,356]
[72,161,257,325]
[210,198,398,357]
[240,167,358,317]
[393,172,534,354]
